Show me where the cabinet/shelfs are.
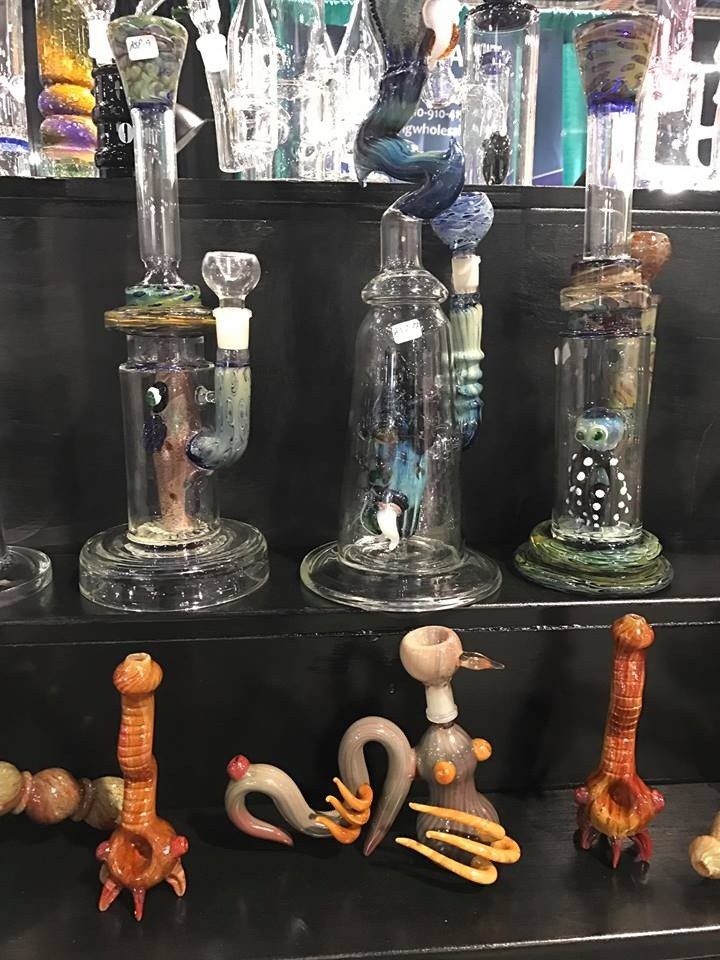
[0,176,720,960]
